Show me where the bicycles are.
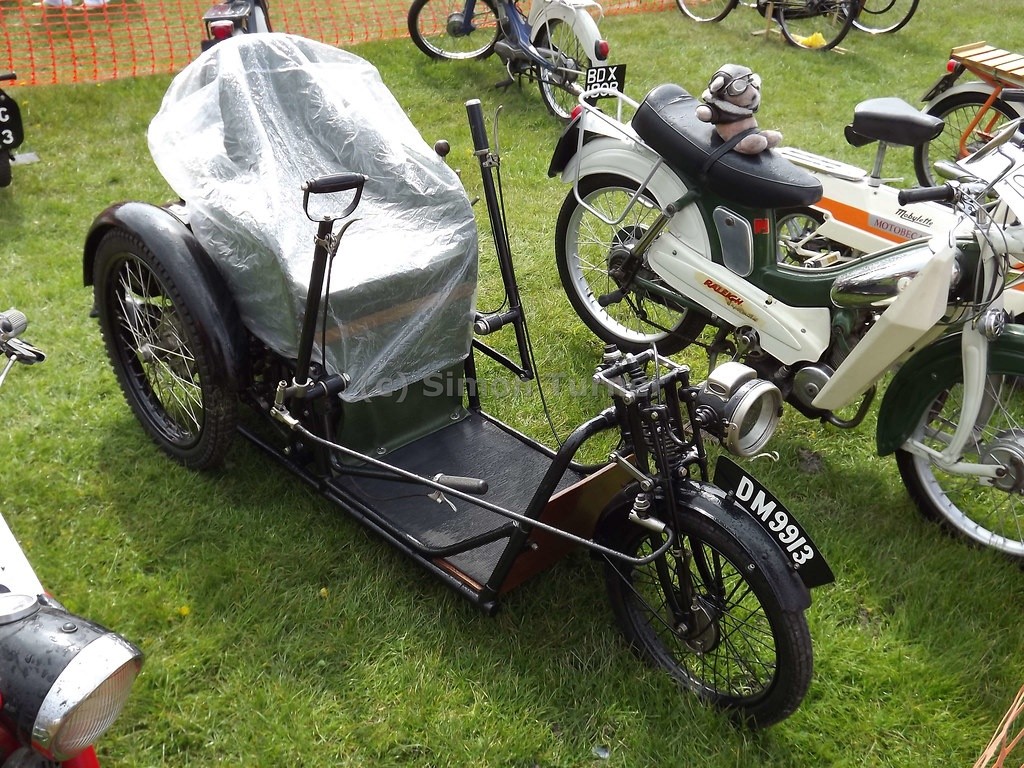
[407,0,614,122]
[835,0,921,35]
[912,40,1024,192]
[197,0,274,59]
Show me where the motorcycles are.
[527,31,1024,563]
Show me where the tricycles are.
[78,31,838,726]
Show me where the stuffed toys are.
[692,63,782,155]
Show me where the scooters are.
[768,94,1024,343]
[0,305,139,766]
[677,0,863,52]
